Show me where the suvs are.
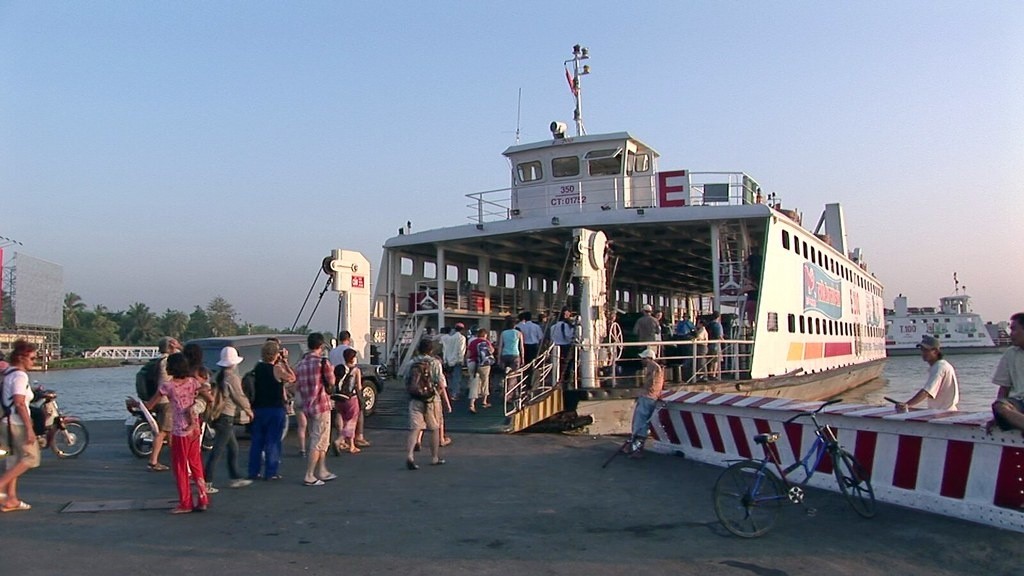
[188,333,384,442]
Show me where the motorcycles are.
[28,379,90,459]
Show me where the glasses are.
[26,355,36,361]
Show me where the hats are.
[643,304,652,312]
[454,322,465,328]
[916,335,940,350]
[639,349,656,360]
[217,347,243,368]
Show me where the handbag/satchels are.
[241,372,257,403]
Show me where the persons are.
[402,339,445,470]
[497,305,726,406]
[293,330,372,458]
[292,333,339,487]
[241,338,297,482]
[895,335,959,412]
[143,351,214,514]
[621,349,665,460]
[0,338,41,512]
[203,346,254,494]
[421,322,467,403]
[991,313,1024,430]
[413,355,452,451]
[466,329,495,414]
[139,336,217,484]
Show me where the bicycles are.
[712,398,878,539]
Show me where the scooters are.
[123,394,216,458]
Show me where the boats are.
[368,43,889,436]
[884,272,996,357]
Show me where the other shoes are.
[350,446,361,453]
[431,457,446,465]
[269,474,284,479]
[480,403,492,409]
[147,462,170,470]
[333,440,342,455]
[451,396,459,401]
[169,507,192,513]
[340,443,349,449]
[230,478,254,487]
[252,473,264,480]
[405,458,419,470]
[356,440,370,447]
[469,406,476,413]
[203,483,220,494]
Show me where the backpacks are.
[207,383,225,420]
[408,355,438,401]
[329,362,358,402]
[0,366,31,420]
[474,341,496,367]
[136,357,165,403]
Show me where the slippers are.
[304,478,326,486]
[322,473,338,481]
[2,499,33,512]
